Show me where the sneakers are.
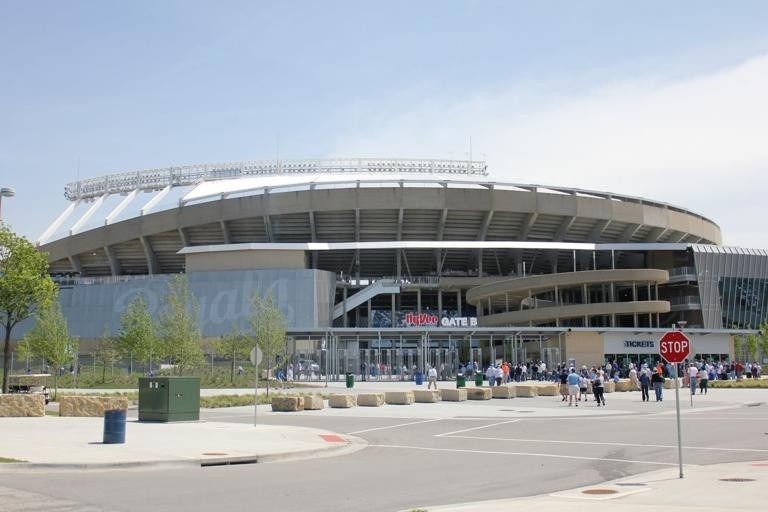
[562,397,605,406]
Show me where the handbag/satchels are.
[594,380,600,387]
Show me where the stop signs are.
[658,328,693,366]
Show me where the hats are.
[643,370,647,373]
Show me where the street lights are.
[0,186,14,213]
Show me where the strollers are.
[547,369,556,382]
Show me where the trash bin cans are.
[457,373,465,389]
[346,371,355,388]
[474,370,484,386]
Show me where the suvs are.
[299,359,320,374]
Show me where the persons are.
[26,364,81,376]
